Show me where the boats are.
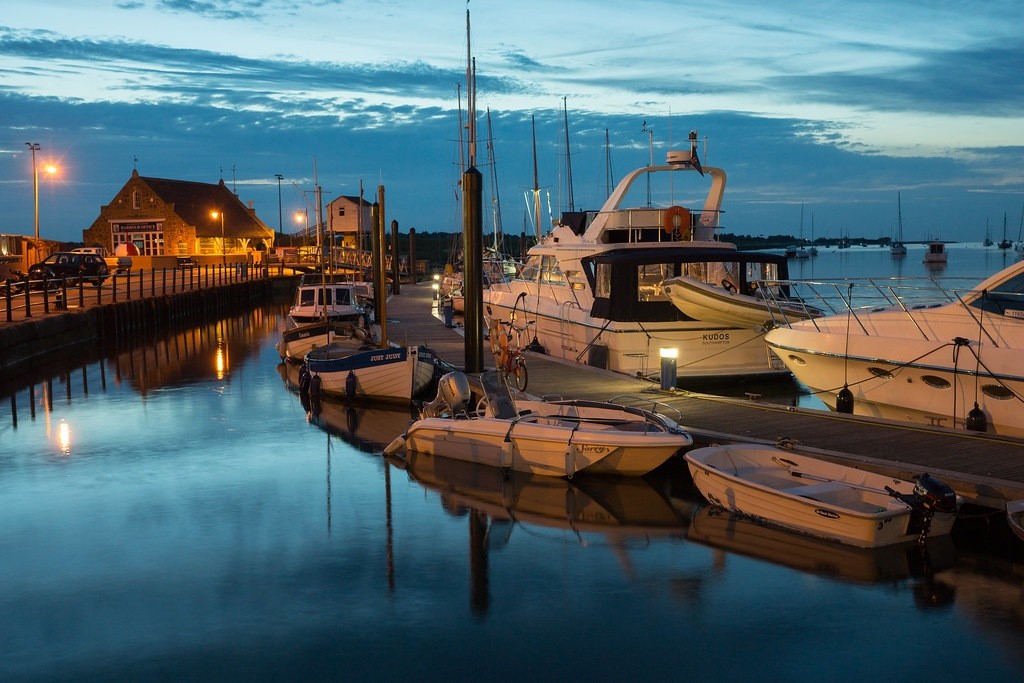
[305,395,424,590]
[923,238,948,263]
[382,450,705,549]
[684,442,964,551]
[660,276,826,330]
[302,185,442,409]
[758,259,1024,439]
[276,186,375,361]
[382,367,694,479]
[483,121,806,387]
[684,504,973,612]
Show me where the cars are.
[70,248,106,257]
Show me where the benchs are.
[778,482,856,507]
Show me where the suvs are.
[28,252,110,287]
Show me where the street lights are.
[211,211,225,265]
[275,174,284,233]
[35,165,58,238]
[25,142,41,176]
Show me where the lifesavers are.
[489,319,508,366]
[663,205,691,236]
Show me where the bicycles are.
[486,320,536,392]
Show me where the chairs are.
[86,258,91,262]
[60,258,66,263]
[74,257,80,262]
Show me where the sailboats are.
[437,0,518,314]
[783,191,1024,259]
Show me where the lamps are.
[660,346,679,359]
[444,299,451,307]
